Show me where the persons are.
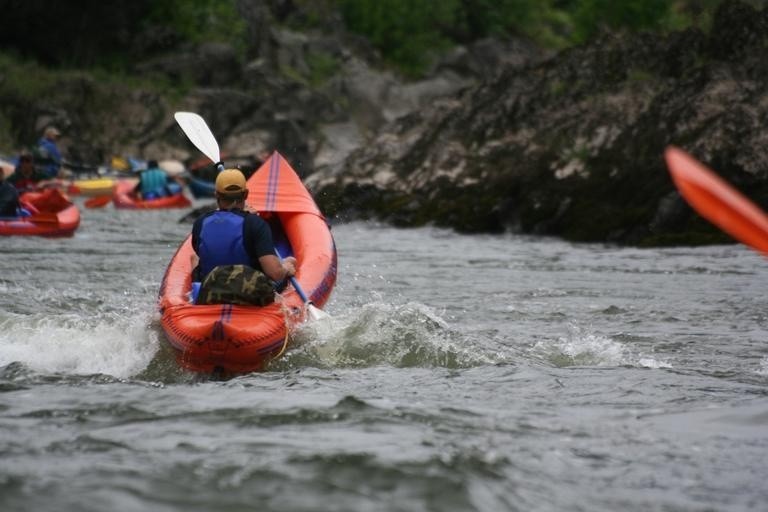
[192,169,297,292]
[0,126,179,220]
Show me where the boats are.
[158,149,338,379]
[0,156,258,239]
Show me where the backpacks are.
[197,264,275,305]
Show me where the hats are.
[215,168,246,195]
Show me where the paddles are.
[73,159,129,209]
[174,111,330,321]
[664,145,768,255]
